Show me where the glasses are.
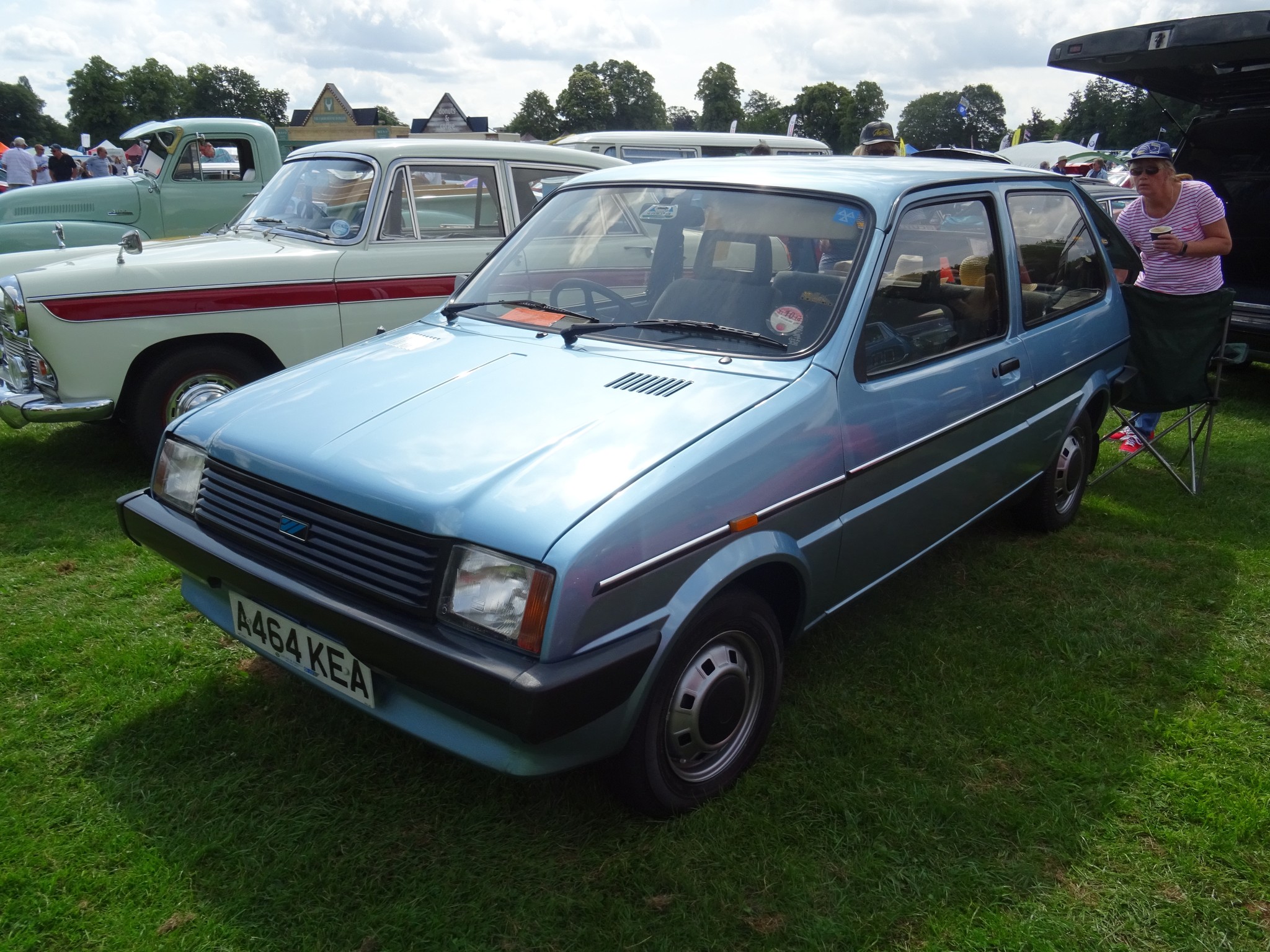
[865,149,896,156]
[106,151,107,154]
[1130,166,1158,176]
[38,149,45,151]
[1048,166,1050,168]
[50,150,56,153]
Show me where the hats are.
[884,254,949,285]
[859,122,901,146]
[1055,155,1070,164]
[14,137,28,147]
[1124,140,1173,162]
[48,144,62,151]
[958,255,1037,315]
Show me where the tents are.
[0,139,142,192]
[464,178,486,188]
[899,144,919,154]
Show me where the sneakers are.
[1102,425,1154,454]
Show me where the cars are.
[894,139,1176,284]
[0,139,795,475]
[114,158,1147,809]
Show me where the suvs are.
[1046,10,1269,376]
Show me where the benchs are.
[773,269,1054,349]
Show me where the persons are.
[1039,161,1050,171]
[411,171,441,186]
[819,122,901,270]
[199,142,235,163]
[749,144,771,156]
[1050,156,1070,175]
[730,120,737,133]
[1086,157,1108,181]
[787,114,797,137]
[33,144,135,189]
[1109,140,1233,453]
[0,137,37,189]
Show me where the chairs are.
[861,240,954,361]
[1088,286,1251,498]
[634,230,782,343]
[243,169,257,181]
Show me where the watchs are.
[1177,241,1188,257]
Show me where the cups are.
[1149,226,1172,248]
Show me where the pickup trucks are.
[0,117,499,254]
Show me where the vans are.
[554,131,832,200]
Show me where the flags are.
[1005,140,1009,148]
[1087,133,1099,150]
[999,135,1008,151]
[1011,129,1020,146]
[1160,127,1166,133]
[1054,134,1059,141]
[1023,129,1031,142]
[1080,137,1084,146]
[957,96,970,116]
[900,138,906,157]
